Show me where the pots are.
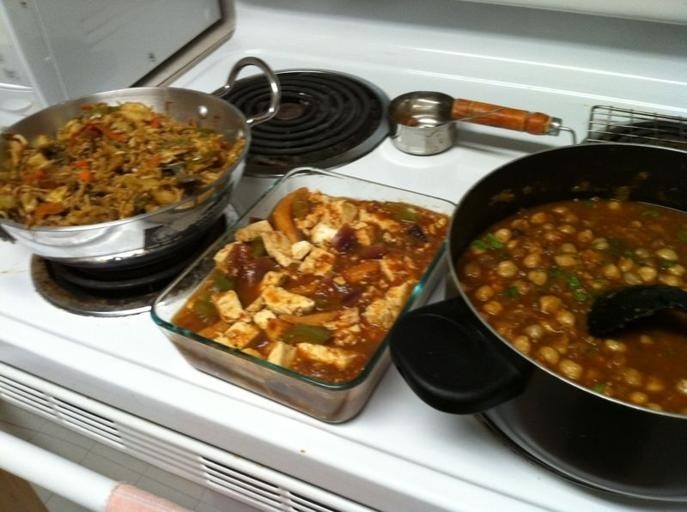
[386,142,687,502]
[1,57,281,269]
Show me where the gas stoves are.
[0,27,687,512]
